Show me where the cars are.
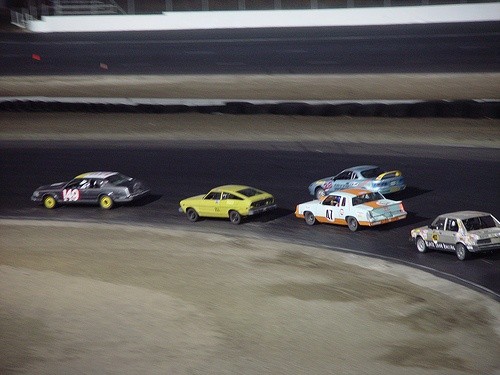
[408,210,500,261]
[178,184,281,225]
[293,189,408,232]
[307,165,408,200]
[30,171,153,210]
